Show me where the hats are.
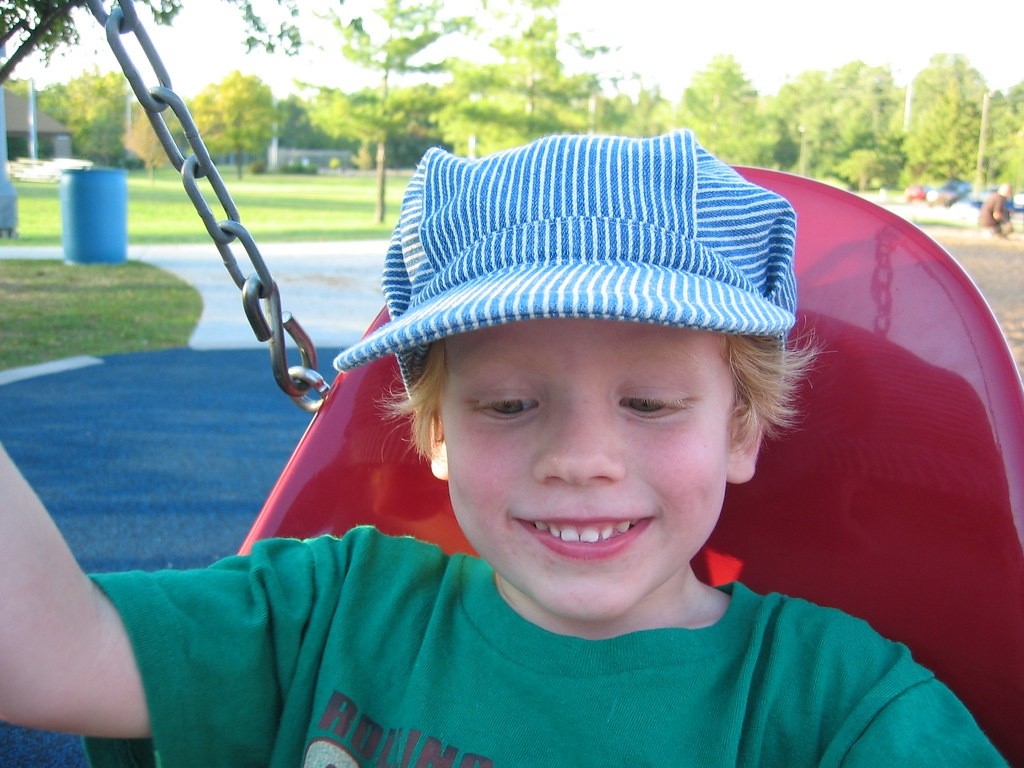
[334,129,798,372]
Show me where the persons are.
[1,129,1012,768]
[978,184,1012,240]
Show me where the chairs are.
[234,164,1024,768]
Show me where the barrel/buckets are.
[61,167,127,266]
